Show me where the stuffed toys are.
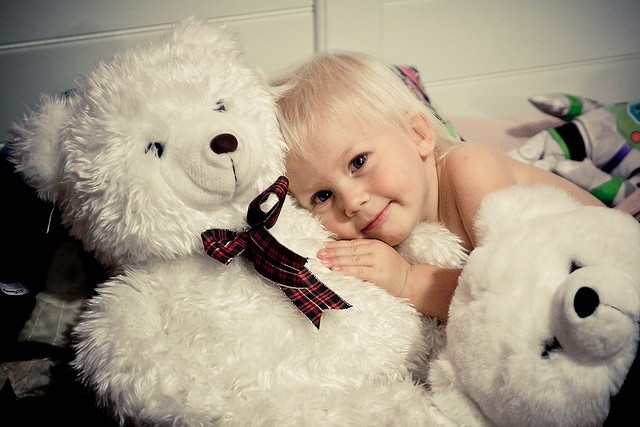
[505,89,639,208]
[10,18,489,426]
[425,183,640,426]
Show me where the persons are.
[277,50,608,330]
